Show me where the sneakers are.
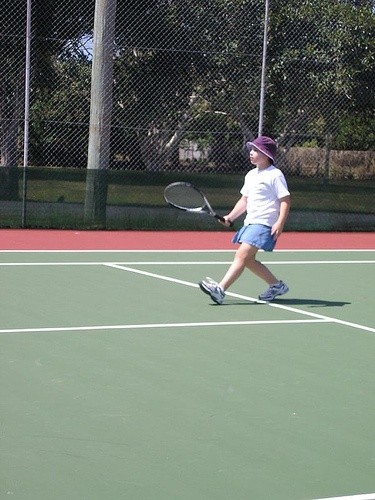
[259,280,289,301]
[199,276,225,305]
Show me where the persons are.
[199,135,290,303]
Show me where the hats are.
[246,136,277,163]
